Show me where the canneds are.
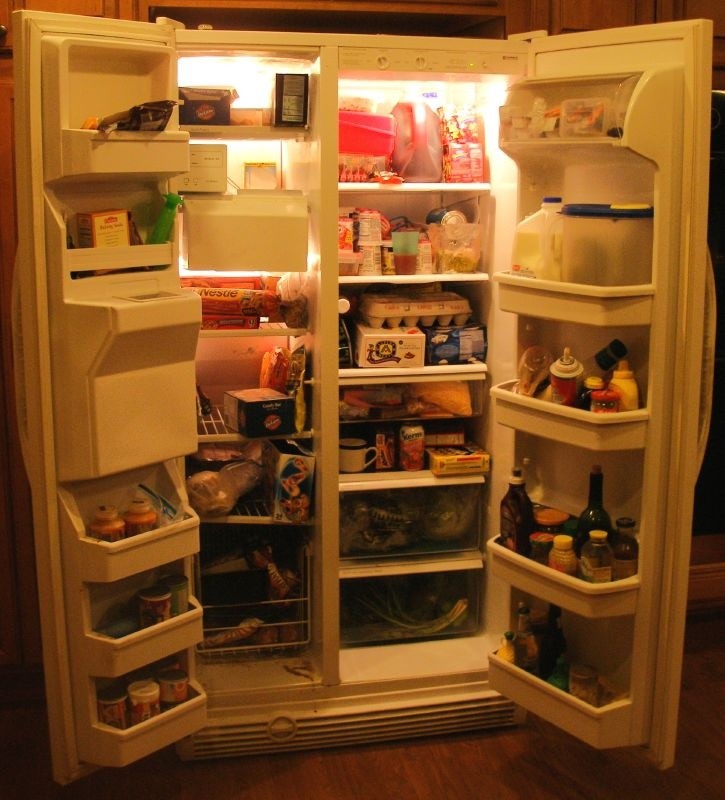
[399,422,425,471]
[136,574,190,629]
[590,390,619,414]
[96,657,188,730]
[567,663,599,707]
[526,508,570,566]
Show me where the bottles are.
[510,196,566,282]
[548,534,578,577]
[612,517,639,581]
[500,601,598,709]
[579,339,629,382]
[500,468,536,558]
[574,463,612,560]
[518,345,553,403]
[89,491,159,542]
[608,360,639,412]
[549,347,584,408]
[578,530,612,584]
[158,655,189,713]
[578,377,604,411]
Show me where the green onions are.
[348,575,470,634]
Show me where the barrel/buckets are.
[560,203,654,286]
[427,222,481,275]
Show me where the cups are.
[339,438,380,474]
[391,232,419,275]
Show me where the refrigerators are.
[8,9,718,786]
